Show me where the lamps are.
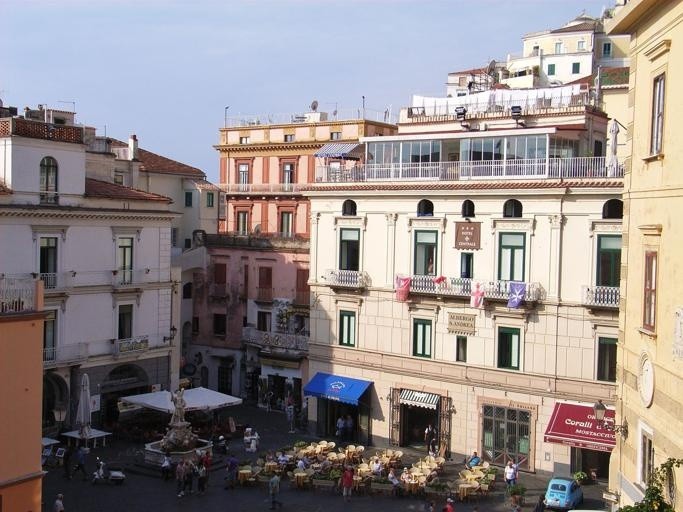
[591,396,628,443]
[162,322,177,345]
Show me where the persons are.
[161,431,211,498]
[466,451,519,490]
[427,258,433,272]
[51,446,89,512]
[222,392,456,512]
[532,494,546,512]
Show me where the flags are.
[507,282,526,309]
[395,275,411,302]
[432,276,447,289]
[470,280,484,308]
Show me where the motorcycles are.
[90,456,127,486]
[208,431,231,454]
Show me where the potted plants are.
[506,484,527,506]
[572,470,588,486]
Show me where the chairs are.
[227,437,504,507]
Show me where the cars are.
[542,475,584,511]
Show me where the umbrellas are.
[75,373,92,455]
